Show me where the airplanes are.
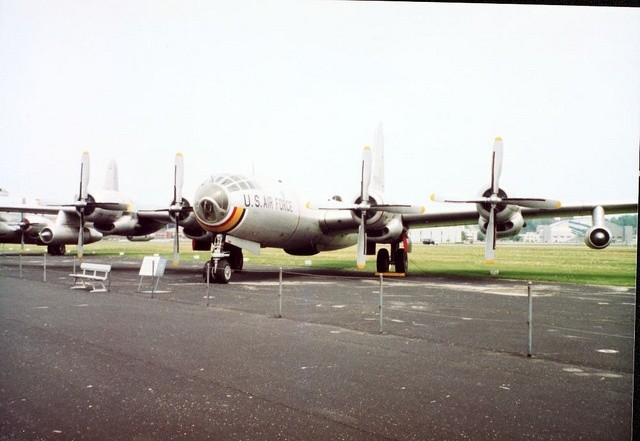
[0,152,638,283]
[1,206,103,256]
